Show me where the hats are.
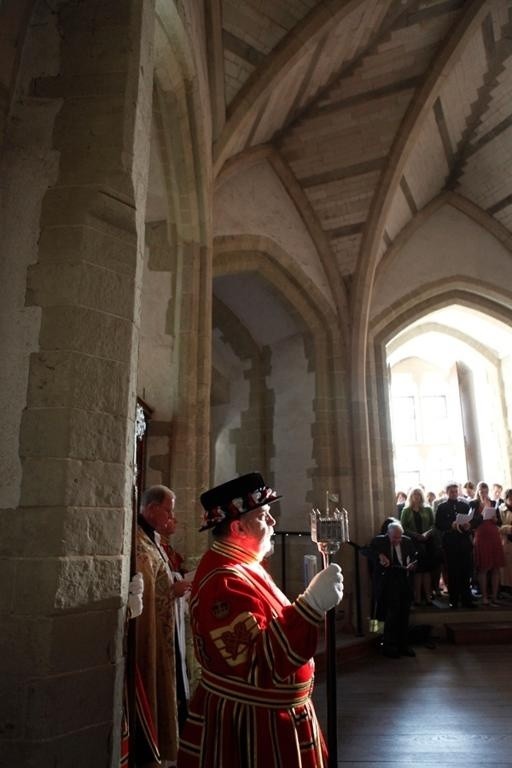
[199,471,284,532]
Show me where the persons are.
[123,569,164,766]
[371,482,511,659]
[175,470,344,768]
[156,514,193,732]
[133,484,178,768]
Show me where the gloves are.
[303,563,345,615]
[128,571,144,619]
